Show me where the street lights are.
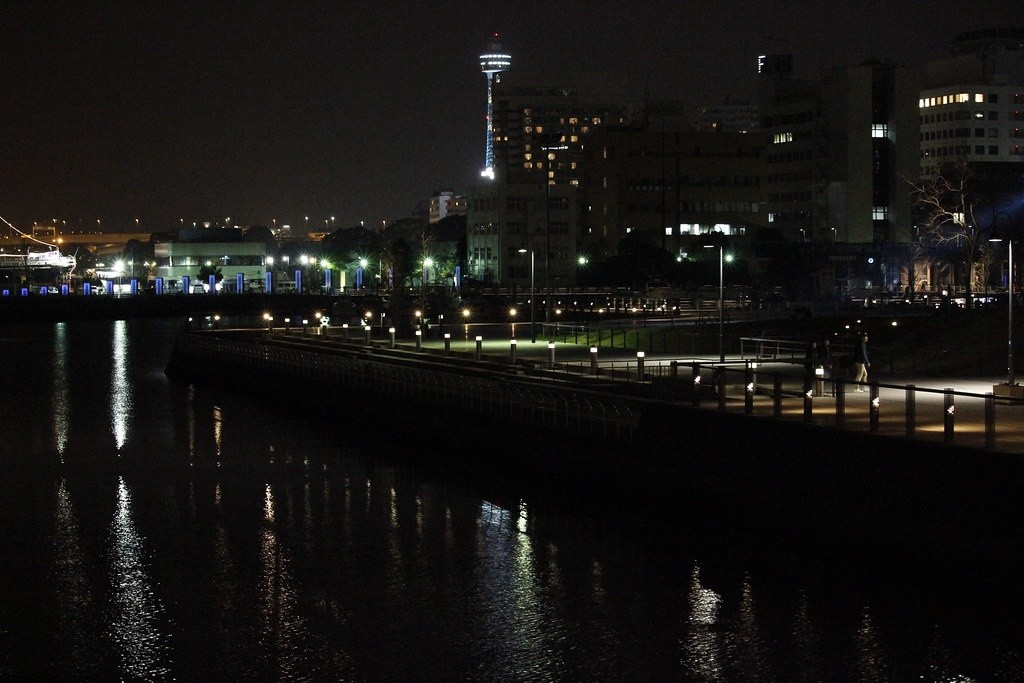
[989,210,1024,403]
[704,224,727,362]
[518,247,538,342]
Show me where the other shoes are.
[862,388,870,391]
[854,389,863,393]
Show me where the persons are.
[853,332,870,393]
[946,284,953,299]
[801,338,833,399]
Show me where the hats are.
[859,333,868,336]
